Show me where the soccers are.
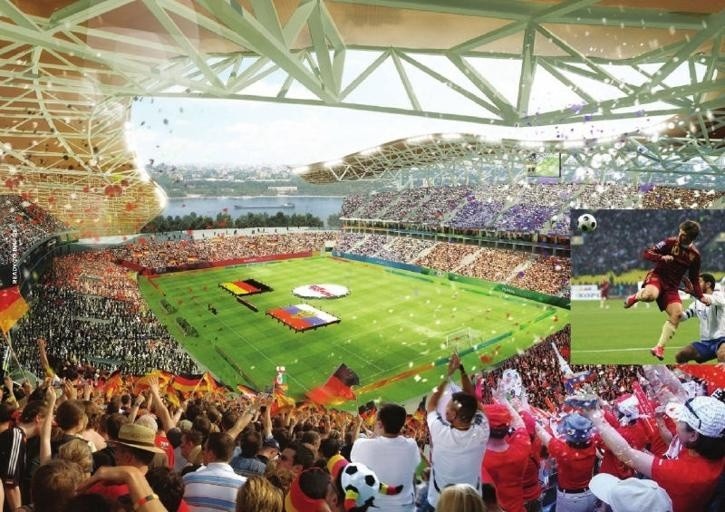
[576,213,598,233]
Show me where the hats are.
[664,394,725,439]
[101,420,170,457]
[555,414,594,445]
[327,453,404,509]
[480,400,514,428]
[282,468,328,511]
[586,472,675,512]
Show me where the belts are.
[558,487,590,494]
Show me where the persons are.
[2,183,725,512]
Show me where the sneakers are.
[621,292,640,310]
[649,344,665,361]
